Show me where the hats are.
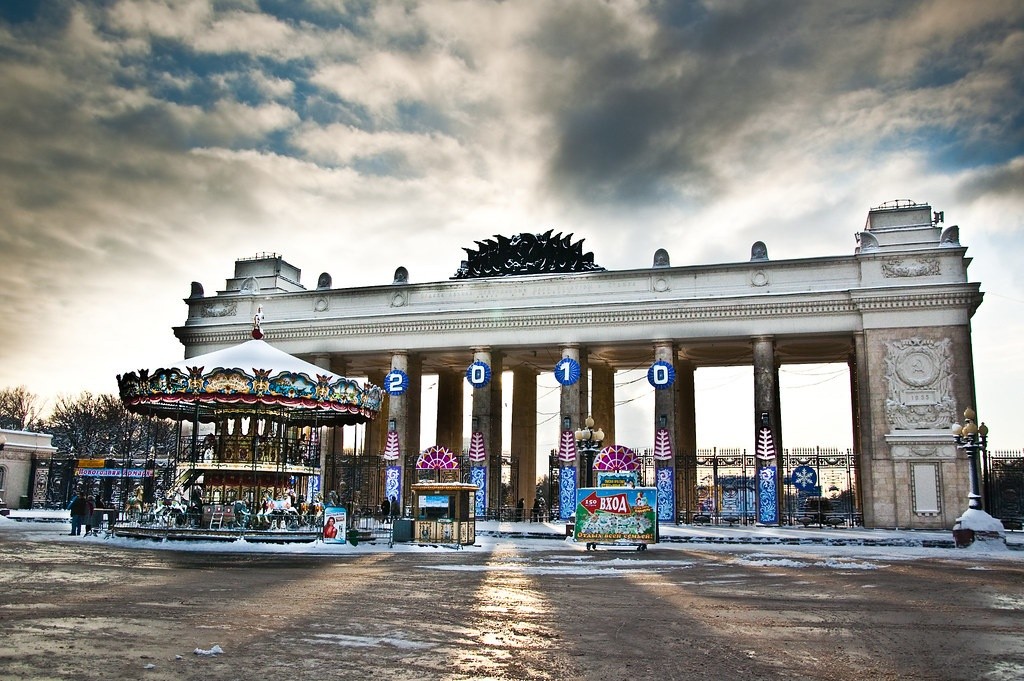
[383,497,388,499]
[95,495,102,500]
[298,495,304,501]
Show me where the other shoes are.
[77,533,80,535]
[68,533,76,535]
[385,522,389,524]
[389,522,392,524]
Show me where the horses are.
[125,485,149,512]
[257,490,299,523]
[314,490,339,526]
[300,492,324,526]
[153,485,187,520]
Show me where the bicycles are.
[126,498,178,525]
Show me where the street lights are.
[951,407,990,546]
[574,417,604,488]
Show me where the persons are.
[516,498,547,522]
[68,486,104,537]
[337,482,348,503]
[381,496,400,524]
